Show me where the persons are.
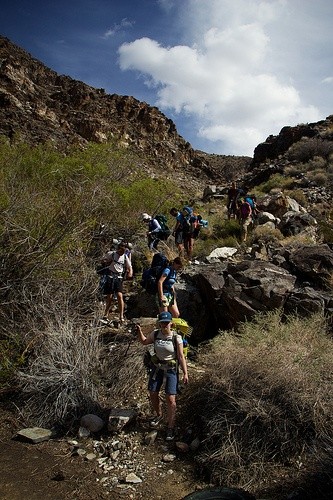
[142,206,208,259]
[99,243,133,324]
[134,312,189,441]
[226,181,257,241]
[155,253,184,318]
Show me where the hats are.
[142,213,151,219]
[158,311,172,322]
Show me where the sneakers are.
[166,428,175,440]
[150,415,164,426]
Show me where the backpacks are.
[151,318,188,382]
[149,214,171,240]
[140,253,176,295]
[107,248,134,280]
